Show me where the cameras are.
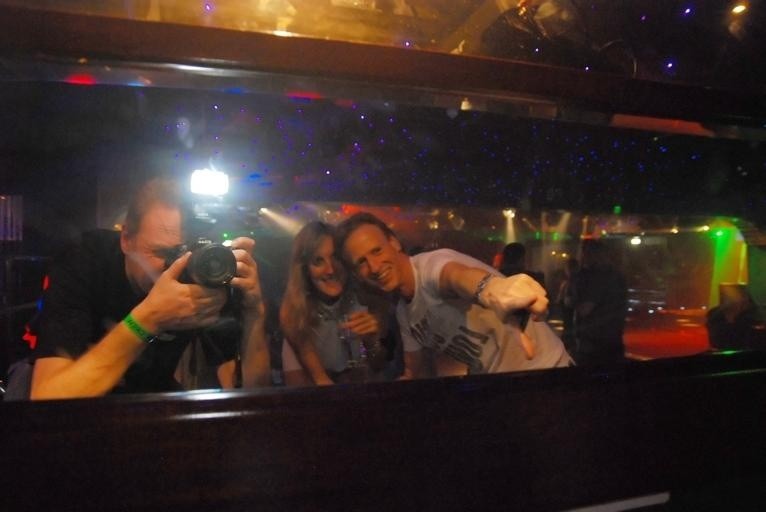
[163,169,235,293]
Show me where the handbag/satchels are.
[3,357,32,401]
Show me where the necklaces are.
[316,288,356,350]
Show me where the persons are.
[3,311,41,402]
[555,256,592,338]
[335,211,577,380]
[278,219,393,386]
[572,237,629,363]
[25,174,273,401]
[497,240,544,287]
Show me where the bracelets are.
[470,271,497,310]
[122,313,159,347]
